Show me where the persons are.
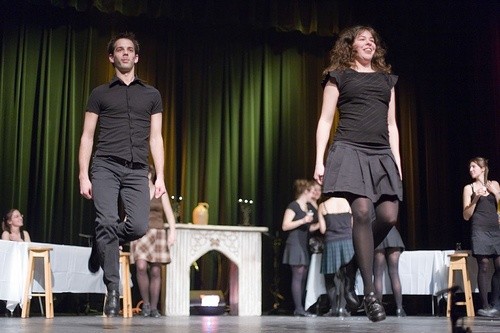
[1,209,31,242]
[464,158,500,317]
[282,181,322,317]
[79,36,166,317]
[373,226,406,317]
[318,197,360,317]
[124,149,175,318]
[314,25,403,322]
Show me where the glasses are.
[14,214,24,219]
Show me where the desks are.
[0,239,133,316]
[163,223,268,315]
[304,250,480,315]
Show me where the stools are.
[447,252,476,317]
[20,246,54,318]
[101,252,133,318]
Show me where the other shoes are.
[325,305,351,317]
[357,302,364,312]
[396,308,406,317]
[478,304,500,319]
[294,309,316,317]
[142,303,162,318]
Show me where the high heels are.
[362,292,386,322]
[336,264,359,306]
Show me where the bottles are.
[192,203,209,226]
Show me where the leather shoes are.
[88,235,101,273]
[105,289,119,316]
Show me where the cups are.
[455,243,461,251]
